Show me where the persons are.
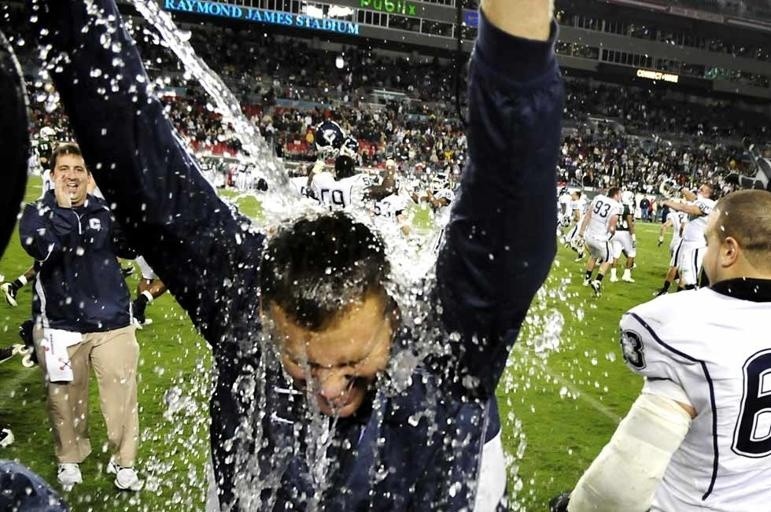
[18,142,147,490]
[551,188,770,510]
[555,18,770,299]
[50,1,565,512]
[1,17,169,368]
[122,18,480,304]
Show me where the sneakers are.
[109,454,139,491]
[1,424,15,449]
[1,282,18,307]
[57,462,82,486]
[0,344,22,364]
[574,253,636,297]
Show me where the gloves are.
[725,172,744,187]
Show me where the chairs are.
[157,95,387,169]
